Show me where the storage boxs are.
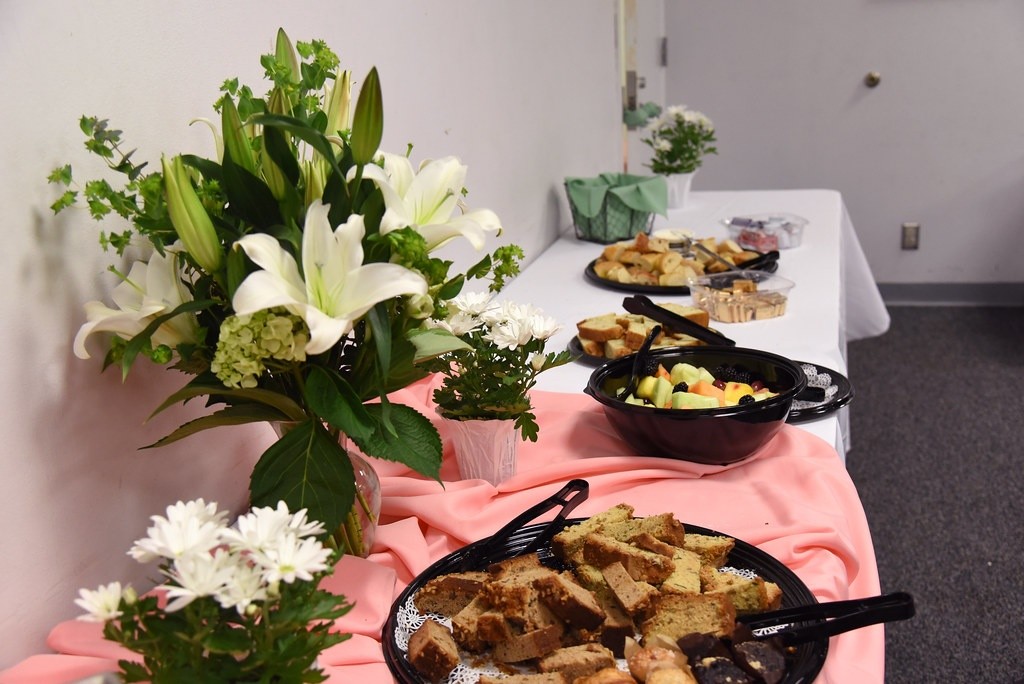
[562,172,670,246]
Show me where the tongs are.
[732,592,917,649]
[622,294,737,347]
[794,383,827,404]
[458,478,590,573]
[724,247,779,273]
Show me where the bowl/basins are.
[582,344,809,466]
[685,267,796,323]
[719,212,809,247]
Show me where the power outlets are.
[900,221,918,252]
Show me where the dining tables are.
[479,185,892,455]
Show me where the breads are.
[593,232,760,286]
[575,302,718,359]
[406,502,786,684]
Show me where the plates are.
[786,359,855,424]
[567,329,722,366]
[381,515,828,684]
[585,239,780,293]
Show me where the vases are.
[434,403,524,488]
[655,170,697,211]
[264,412,384,560]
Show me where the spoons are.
[610,324,665,401]
[684,234,760,280]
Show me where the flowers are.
[638,105,720,180]
[67,497,356,684]
[417,290,585,447]
[42,20,588,546]
